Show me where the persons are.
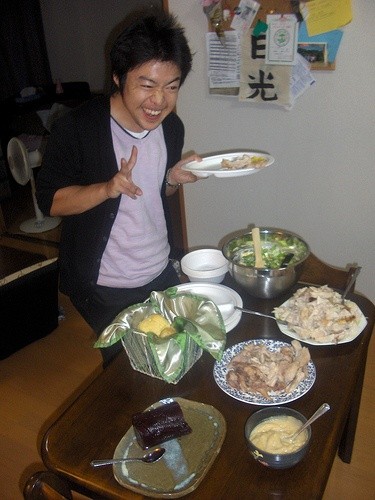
[32,11,206,368]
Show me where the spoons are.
[89,447,166,468]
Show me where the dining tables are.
[23,281,375,500]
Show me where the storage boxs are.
[120,291,212,385]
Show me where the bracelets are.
[165,167,184,189]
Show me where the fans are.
[7,137,61,233]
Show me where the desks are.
[12,82,91,136]
[0,230,65,362]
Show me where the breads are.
[131,401,192,450]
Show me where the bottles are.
[56,80,64,94]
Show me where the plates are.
[164,282,243,334]
[180,151,276,177]
[213,338,316,406]
[275,297,367,346]
[112,397,226,500]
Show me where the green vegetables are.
[237,239,299,269]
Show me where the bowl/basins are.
[181,249,229,284]
[116,298,204,384]
[221,227,311,299]
[245,407,312,470]
